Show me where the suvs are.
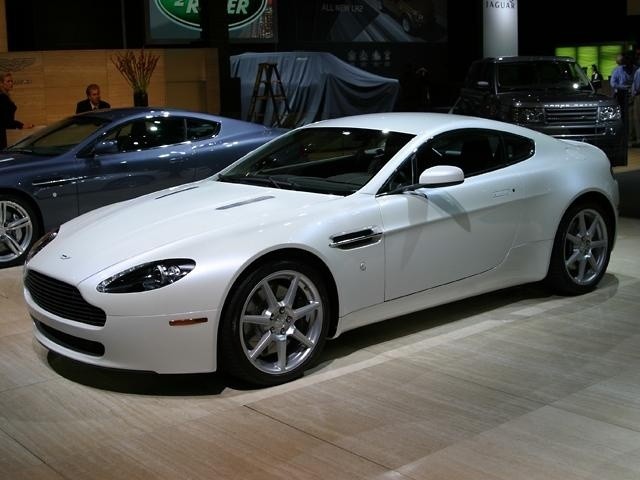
[462,57,627,166]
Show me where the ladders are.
[246,62,295,127]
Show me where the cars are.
[23,112,621,385]
[0,107,292,269]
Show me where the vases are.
[133,86,149,107]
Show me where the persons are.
[590,64,604,85]
[76,83,111,112]
[1,71,34,151]
[610,53,640,149]
